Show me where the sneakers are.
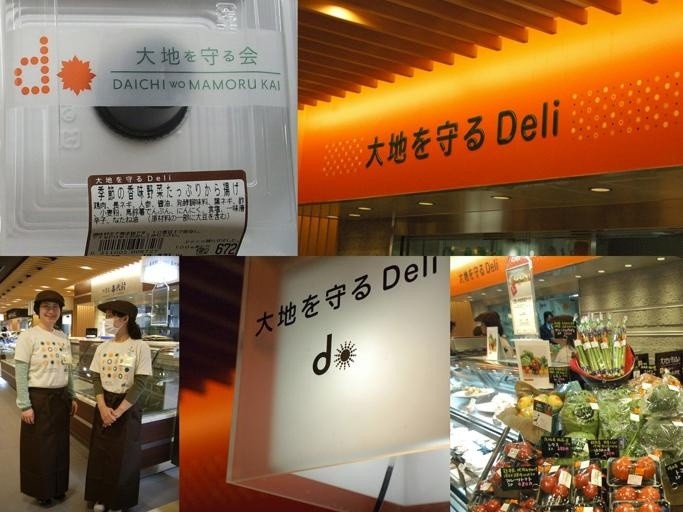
[108,508,122,512]
[93,500,106,512]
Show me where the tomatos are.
[472,442,665,512]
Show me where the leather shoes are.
[53,492,65,500]
[38,500,52,508]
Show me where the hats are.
[35,290,64,306]
[474,313,500,323]
[98,300,138,319]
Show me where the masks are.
[104,319,123,335]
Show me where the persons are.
[14,289,78,508]
[450,321,457,356]
[474,312,511,353]
[84,300,153,511]
[540,311,560,343]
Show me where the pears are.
[516,393,562,419]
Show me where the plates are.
[455,387,494,397]
[475,404,495,418]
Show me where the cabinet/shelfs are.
[0,328,180,479]
[450,355,562,512]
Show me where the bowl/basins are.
[569,343,636,382]
